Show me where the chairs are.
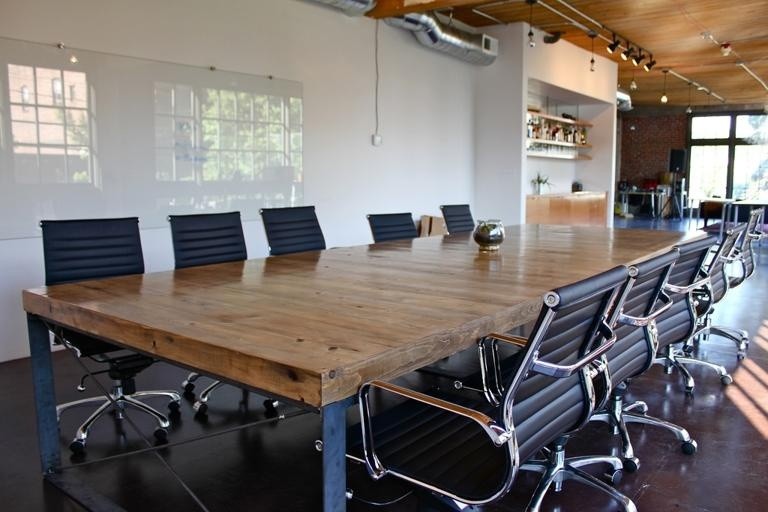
[167,211,280,419]
[451,250,698,474]
[38,216,182,454]
[259,205,326,257]
[651,234,731,395]
[314,264,634,512]
[730,208,765,288]
[707,222,748,363]
[366,212,418,244]
[440,204,475,234]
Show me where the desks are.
[20,224,712,512]
[620,190,672,218]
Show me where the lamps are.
[632,48,645,67]
[528,2,536,48]
[621,40,634,61]
[630,68,637,92]
[644,54,657,72]
[607,33,620,54]
[661,71,669,103]
[686,84,692,114]
[588,30,598,72]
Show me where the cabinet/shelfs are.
[526,104,614,227]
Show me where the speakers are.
[668,147,685,174]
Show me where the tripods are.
[658,176,683,220]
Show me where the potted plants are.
[530,172,552,195]
